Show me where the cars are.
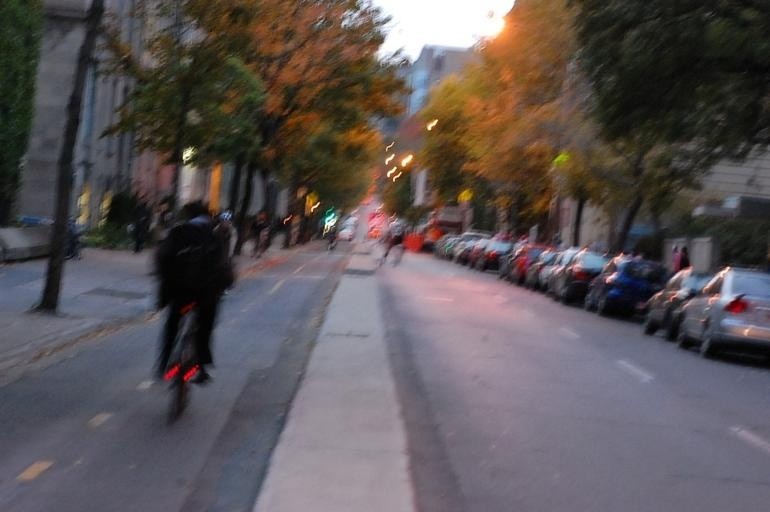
[645,267,716,341]
[676,265,770,359]
[432,231,670,322]
[336,203,383,246]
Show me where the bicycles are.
[164,307,210,424]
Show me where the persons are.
[64,201,405,258]
[155,201,233,383]
[672,245,689,274]
